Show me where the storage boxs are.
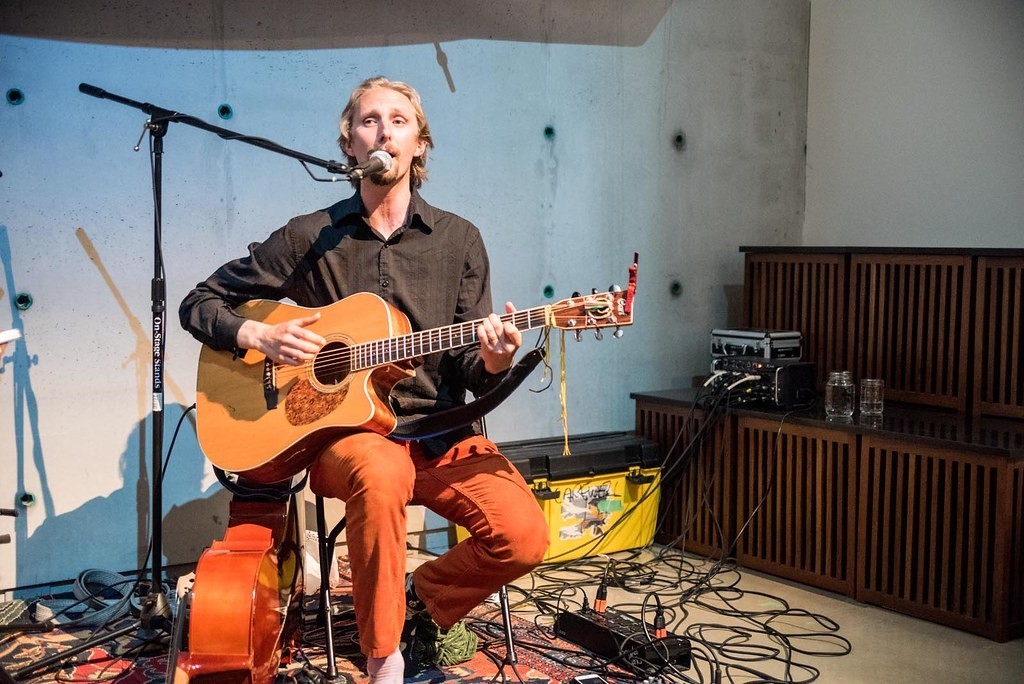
[455,432,663,563]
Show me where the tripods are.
[8,83,372,680]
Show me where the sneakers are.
[398,571,446,684]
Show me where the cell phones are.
[574,673,609,684]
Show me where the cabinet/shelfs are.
[630,246,1024,643]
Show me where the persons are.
[179,77,551,684]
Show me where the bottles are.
[823,370,855,417]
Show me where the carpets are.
[0,555,679,684]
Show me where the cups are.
[859,378,884,415]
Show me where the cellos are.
[164,469,307,684]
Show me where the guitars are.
[195,284,634,484]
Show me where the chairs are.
[317,348,548,677]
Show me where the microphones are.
[346,150,392,182]
